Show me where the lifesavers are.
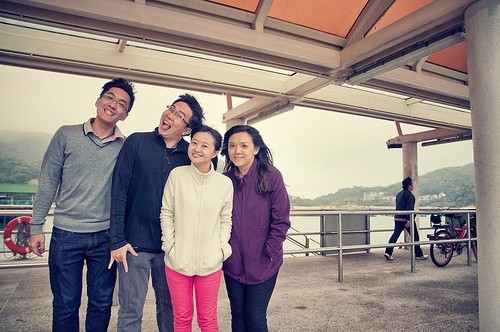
[3,215,43,255]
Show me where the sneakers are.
[415,254,429,260]
[384,253,393,260]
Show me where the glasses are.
[166,105,189,126]
[101,93,128,114]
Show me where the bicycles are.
[427,214,478,267]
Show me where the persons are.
[384,176,429,260]
[29,78,137,332]
[220,125,291,332]
[160,124,233,332]
[108,93,206,332]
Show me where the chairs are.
[427,213,451,250]
